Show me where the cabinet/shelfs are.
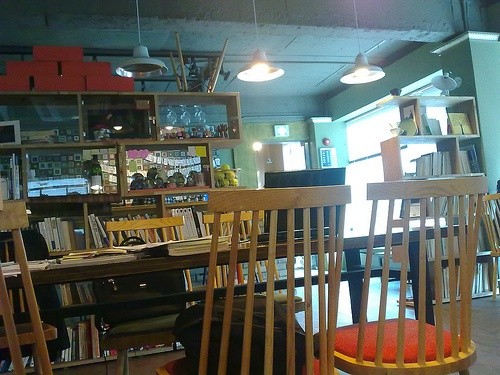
[372,93,500,306]
[0,86,244,375]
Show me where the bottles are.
[187,195,205,203]
[91,154,103,191]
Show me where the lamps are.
[431,70,462,91]
[339,0,386,85]
[236,0,286,83]
[115,0,169,79]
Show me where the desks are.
[0,214,477,340]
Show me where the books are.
[1,236,231,272]
[38,217,76,252]
[0,321,92,373]
[167,206,210,240]
[88,214,161,248]
[100,324,118,357]
[416,145,479,176]
[427,195,491,299]
[56,281,94,305]
[216,263,244,287]
[422,113,473,135]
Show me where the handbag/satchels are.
[93,237,189,333]
[173,295,306,375]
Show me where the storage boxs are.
[0,45,135,91]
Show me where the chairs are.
[203,213,305,313]
[154,182,354,375]
[0,230,59,375]
[0,200,57,375]
[99,215,196,375]
[313,171,490,375]
